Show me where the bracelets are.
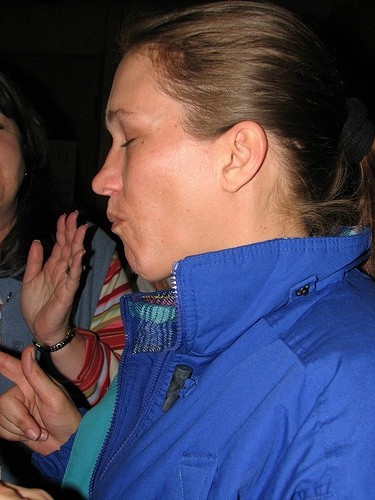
[32,327,76,353]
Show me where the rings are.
[66,265,85,275]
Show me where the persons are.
[0,0,375,500]
